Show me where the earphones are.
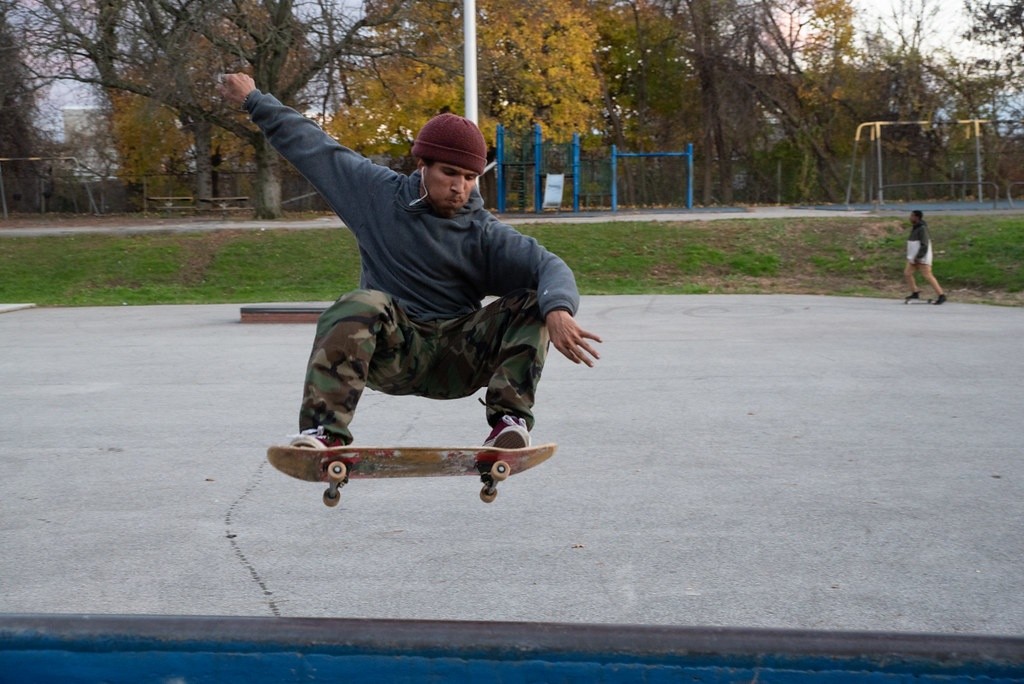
[421,166,425,179]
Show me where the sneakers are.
[290,430,343,451]
[480,419,531,457]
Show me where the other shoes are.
[905,293,919,299]
[933,295,946,305]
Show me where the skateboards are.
[266,443,558,507]
[903,296,937,305]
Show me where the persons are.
[215,72,603,449]
[903,209,947,305]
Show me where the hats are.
[413,112,486,175]
